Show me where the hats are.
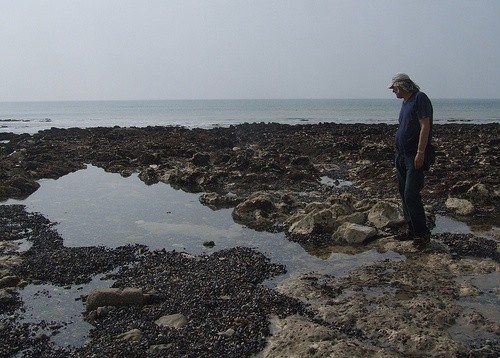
[387,72,410,89]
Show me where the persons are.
[386,72,433,245]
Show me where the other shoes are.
[394,230,415,240]
[412,231,431,245]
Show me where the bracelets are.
[416,150,425,154]
